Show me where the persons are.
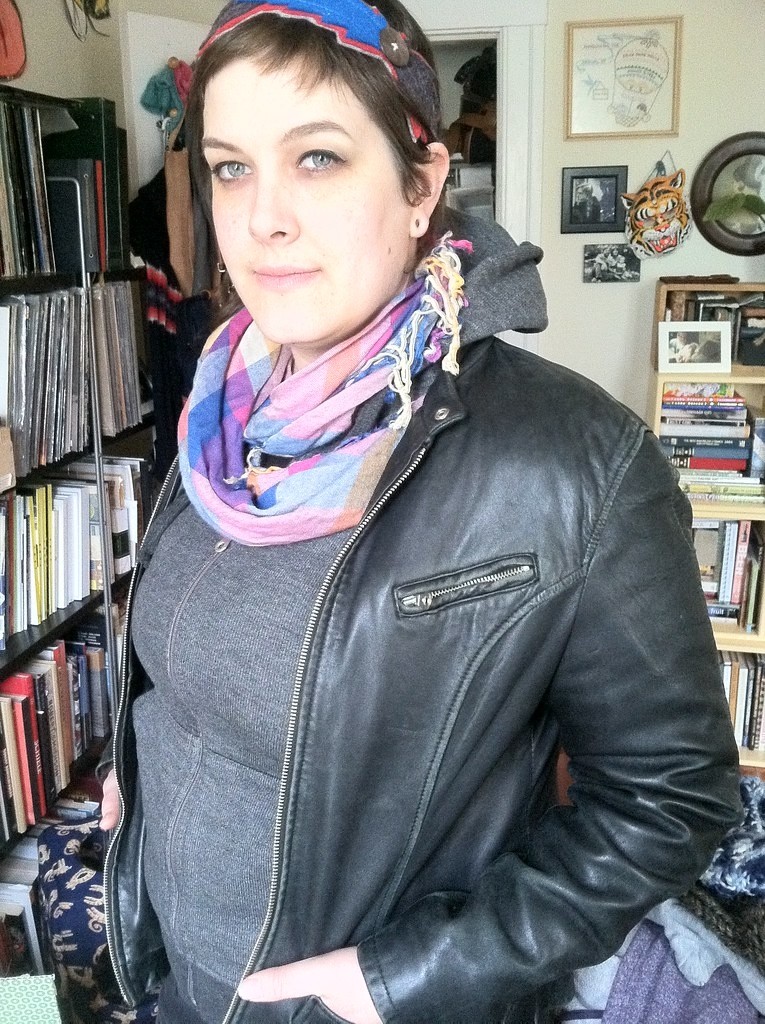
[91,0,743,1024]
[571,182,601,222]
[670,331,699,363]
[589,247,633,283]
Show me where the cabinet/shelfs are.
[644,281,765,776]
[0,85,180,1024]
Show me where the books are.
[658,383,765,503]
[0,596,128,845]
[0,453,154,649]
[0,777,101,999]
[0,282,143,494]
[690,518,764,633]
[0,96,123,279]
[716,650,765,751]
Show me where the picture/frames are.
[560,165,629,234]
[690,131,765,256]
[564,15,683,141]
[658,321,731,374]
